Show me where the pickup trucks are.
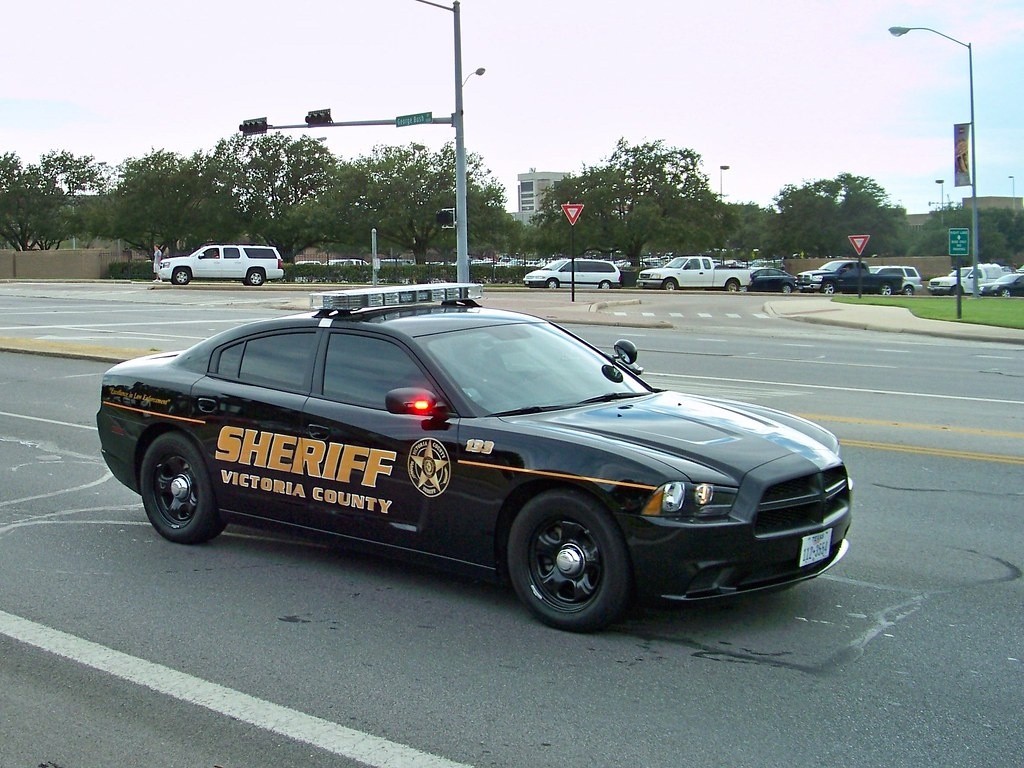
[796,259,903,296]
[636,256,750,293]
[927,266,997,297]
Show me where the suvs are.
[158,244,286,287]
[867,265,924,297]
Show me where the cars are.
[524,257,621,291]
[97,282,854,634]
[981,262,1024,285]
[748,265,800,295]
[295,253,786,274]
[978,273,1024,297]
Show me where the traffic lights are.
[239,117,267,135]
[436,207,456,229]
[305,108,332,126]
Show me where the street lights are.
[889,24,981,298]
[1009,176,1016,209]
[720,165,730,202]
[454,66,486,285]
[935,180,944,224]
